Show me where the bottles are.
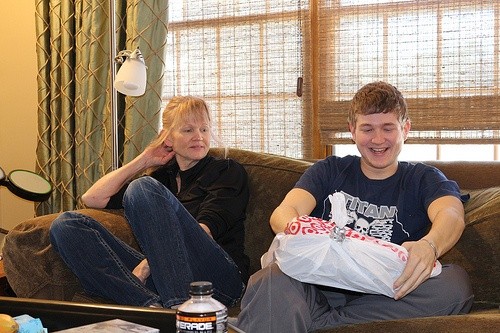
[175,281,229,333]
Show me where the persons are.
[47,95,248,316]
[236,81,473,332]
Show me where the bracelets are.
[421,238,439,261]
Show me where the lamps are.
[111,48,149,97]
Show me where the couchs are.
[2,146,499,333]
